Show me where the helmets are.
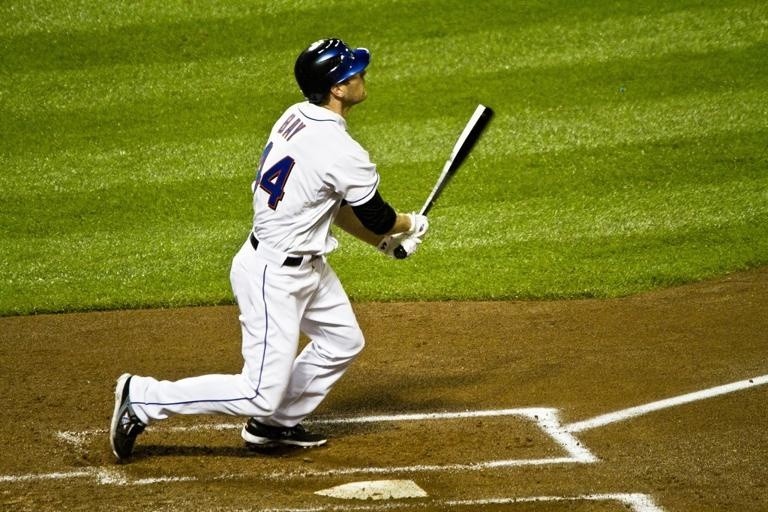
[294,38,370,97]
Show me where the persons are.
[109,36,430,464]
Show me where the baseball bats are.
[393,104,495,259]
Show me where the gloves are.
[376,212,429,257]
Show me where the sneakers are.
[242,416,328,447]
[110,373,149,463]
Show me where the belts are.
[249,232,322,266]
